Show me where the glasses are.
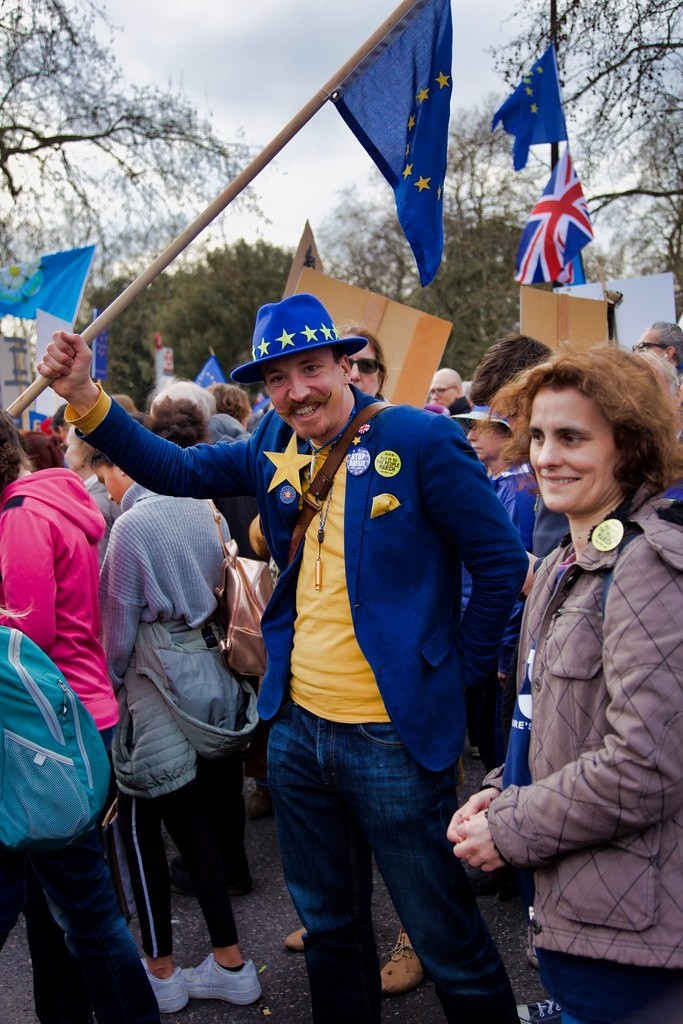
[430,384,456,395]
[348,358,383,374]
[632,342,668,351]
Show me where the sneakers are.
[516,997,562,1024]
[181,952,263,1004]
[140,958,189,1012]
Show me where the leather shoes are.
[285,927,308,949]
[380,930,424,992]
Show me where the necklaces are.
[308,452,334,590]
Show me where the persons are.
[429,324,683,1024]
[249,329,423,995]
[38,294,529,1024]
[447,347,682,1024]
[1,382,260,1024]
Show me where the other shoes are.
[463,864,502,897]
[527,947,539,966]
[167,855,254,895]
[249,784,272,819]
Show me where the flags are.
[333,0,453,288]
[0,244,94,322]
[195,358,225,388]
[514,149,594,286]
[491,44,568,171]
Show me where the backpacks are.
[0,625,110,850]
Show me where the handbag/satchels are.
[207,498,273,677]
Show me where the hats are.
[229,293,368,384]
[108,394,142,418]
[423,403,451,417]
[450,402,511,428]
